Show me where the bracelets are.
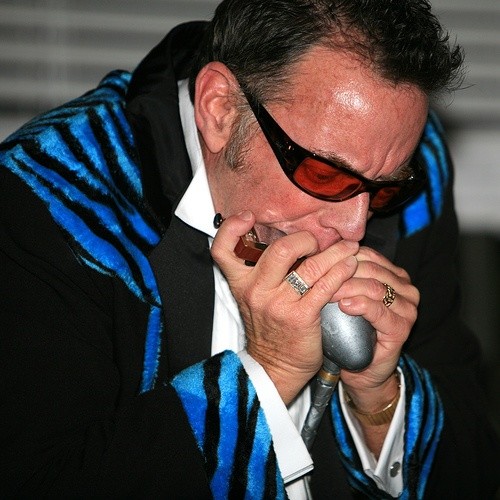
[343,372,402,427]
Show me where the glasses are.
[239,79,424,213]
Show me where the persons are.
[0,0,500,500]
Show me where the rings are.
[381,283,395,308]
[286,272,309,297]
